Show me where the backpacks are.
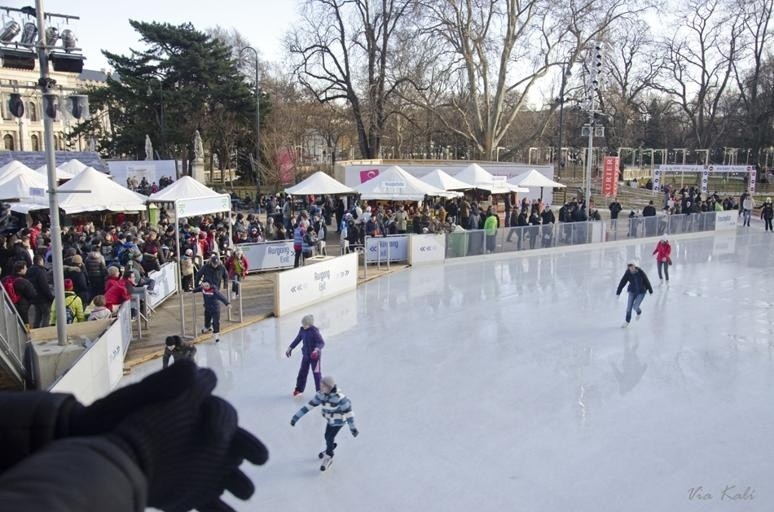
[1,275,22,304]
[66,295,78,324]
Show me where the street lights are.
[1,0,91,348]
[235,42,263,193]
[528,147,538,165]
[560,147,569,168]
[496,147,506,162]
[616,147,773,190]
[583,41,604,222]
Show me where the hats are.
[302,314,314,325]
[320,376,336,395]
[71,254,82,263]
[185,249,193,256]
[211,256,219,265]
[201,280,210,289]
[108,266,119,276]
[65,279,73,291]
[22,229,32,236]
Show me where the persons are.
[0,175,245,344]
[615,260,652,329]
[290,376,360,471]
[742,194,757,227]
[163,334,197,368]
[761,203,774,232]
[652,234,672,288]
[245,177,739,268]
[0,360,268,511]
[738,189,750,218]
[756,197,772,224]
[284,314,325,396]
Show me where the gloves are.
[310,348,320,359]
[290,419,295,426]
[285,346,292,357]
[351,429,359,437]
[70,359,269,511]
[94,368,244,510]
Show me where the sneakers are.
[293,391,300,396]
[201,327,211,333]
[320,456,333,471]
[215,333,220,342]
[318,451,326,458]
